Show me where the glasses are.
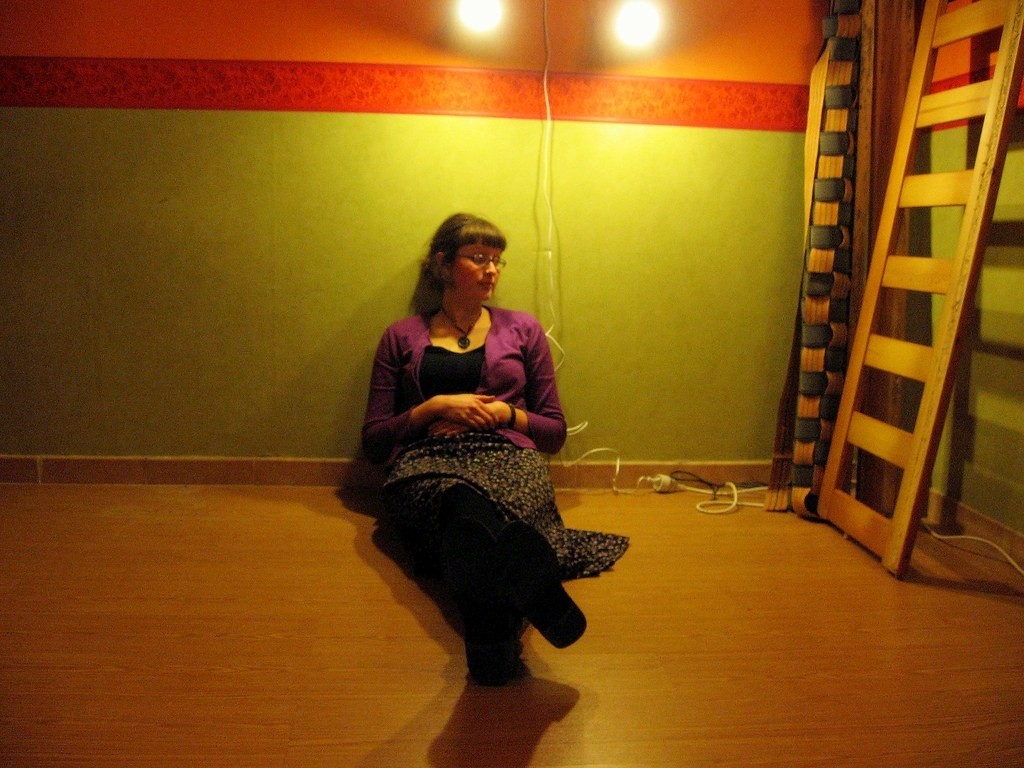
[455,252,507,272]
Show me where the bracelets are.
[506,402,516,429]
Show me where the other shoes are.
[495,522,586,649]
[463,596,521,686]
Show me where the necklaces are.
[440,306,483,349]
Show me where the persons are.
[363,211,631,690]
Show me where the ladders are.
[815,0,1024,578]
